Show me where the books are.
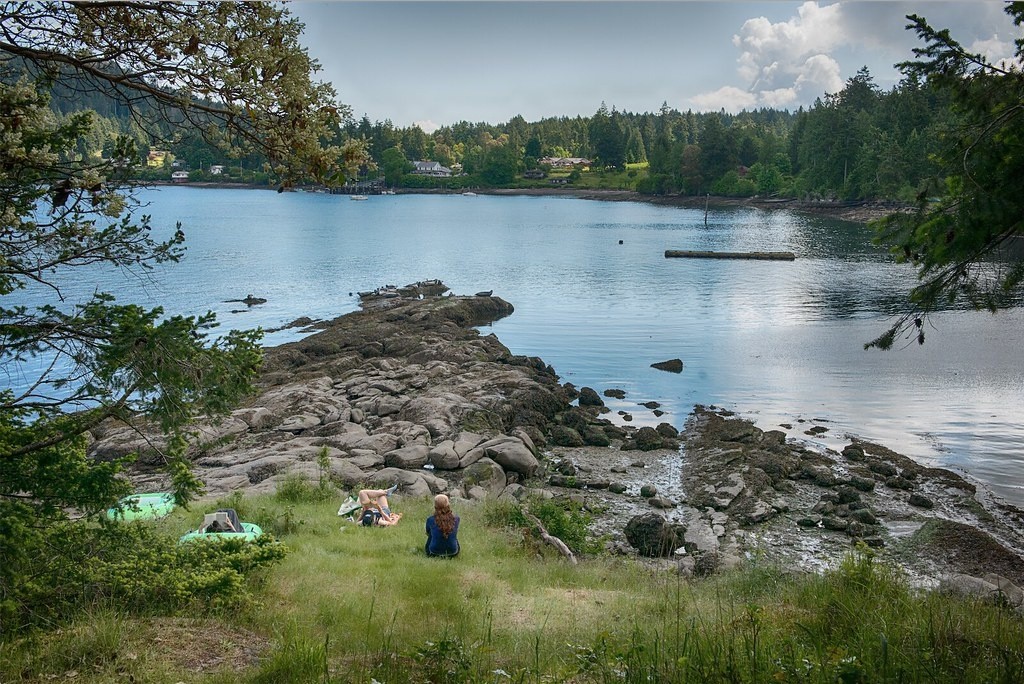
[205,512,227,528]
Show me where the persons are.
[357,485,397,526]
[198,508,244,535]
[425,494,460,560]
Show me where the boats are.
[462,191,477,197]
[350,195,368,200]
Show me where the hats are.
[363,510,374,525]
